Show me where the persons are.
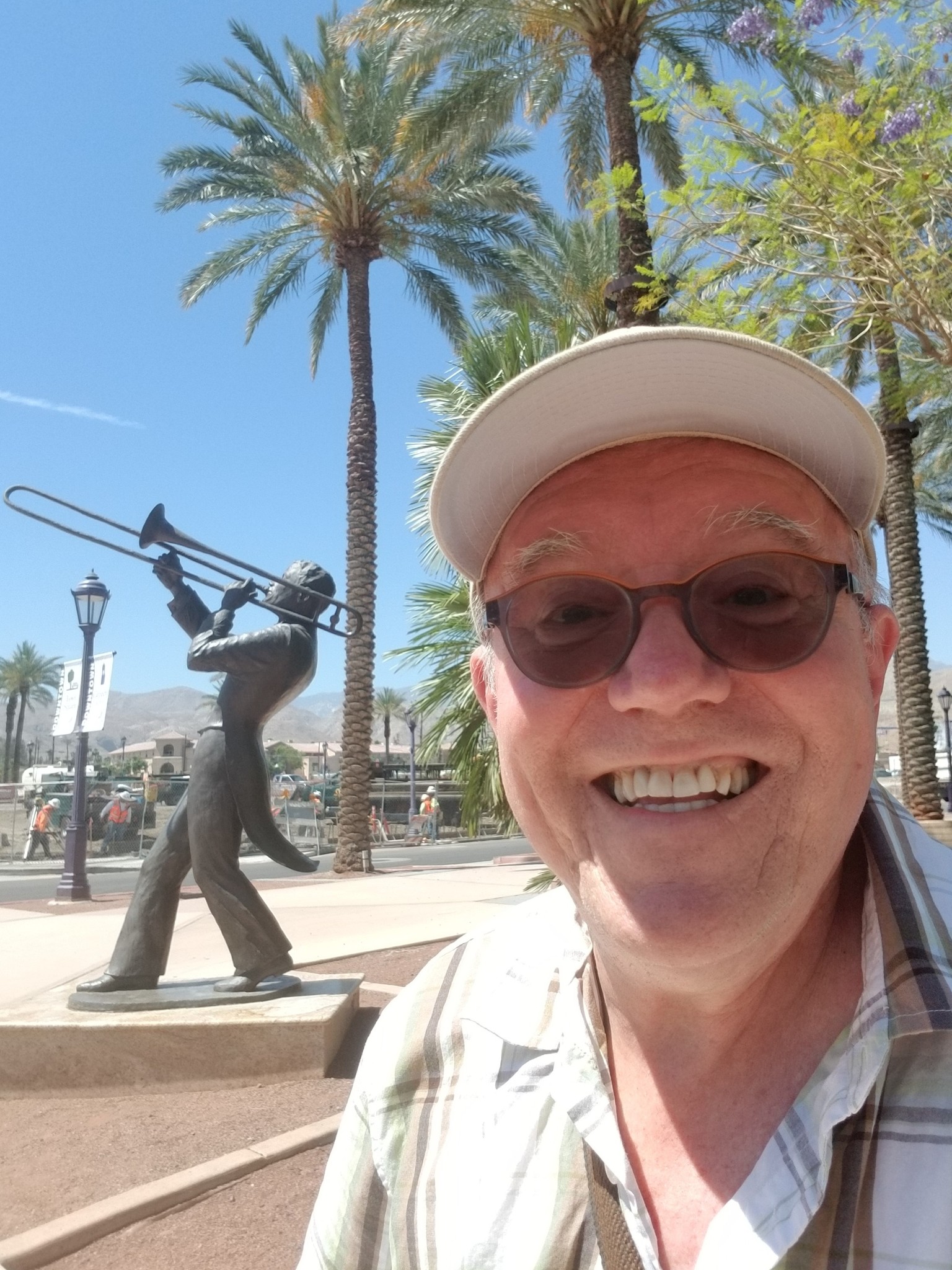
[74,552,336,991]
[420,786,443,841]
[309,790,326,838]
[28,798,61,860]
[100,791,134,856]
[292,328,952,1270]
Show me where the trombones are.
[1,482,363,638]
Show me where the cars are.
[269,761,491,827]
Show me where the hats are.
[120,790,137,802]
[431,325,889,583]
[48,798,60,808]
[313,791,321,798]
[426,785,436,792]
[420,794,430,802]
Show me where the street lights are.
[26,739,35,768]
[404,703,424,847]
[321,740,329,819]
[120,735,126,777]
[44,565,114,903]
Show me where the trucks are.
[13,761,191,841]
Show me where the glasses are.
[475,550,872,684]
